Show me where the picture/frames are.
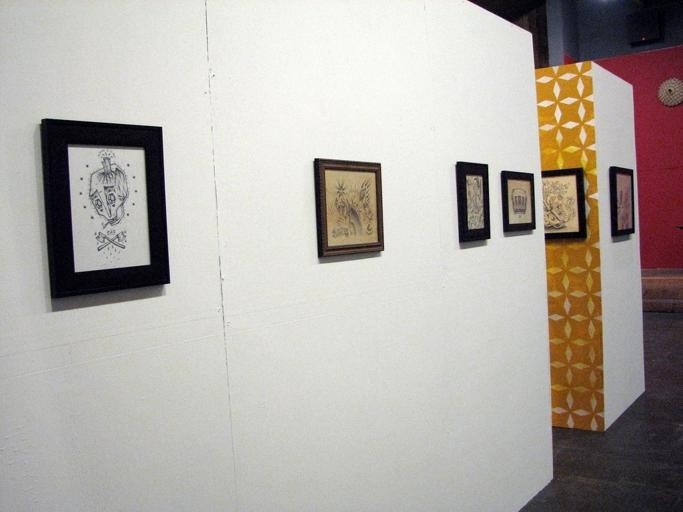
[455,160,534,242]
[541,167,634,240]
[310,158,384,258]
[38,116,170,297]
